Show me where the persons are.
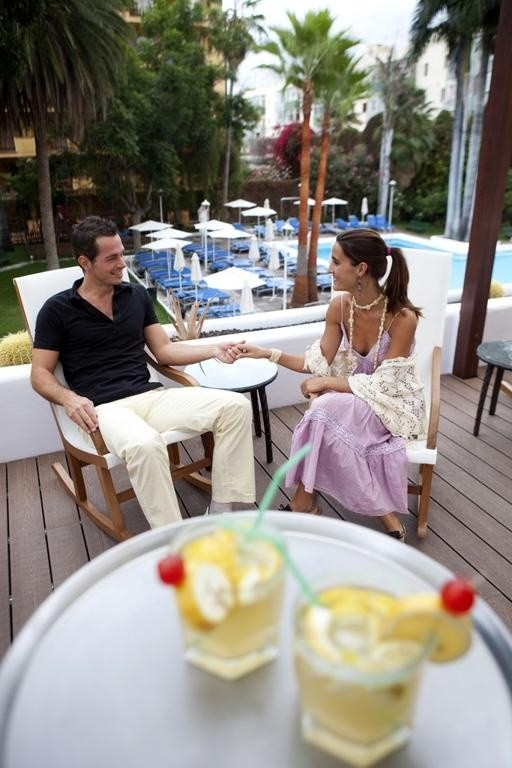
[235,231,428,544]
[24,214,271,530]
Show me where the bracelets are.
[267,347,282,365]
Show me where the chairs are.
[332,241,454,540]
[14,243,213,542]
[132,214,395,318]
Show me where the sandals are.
[387,520,406,543]
[277,504,322,514]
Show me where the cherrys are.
[157,551,184,586]
[439,580,473,612]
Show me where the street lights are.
[158,186,164,222]
[280,222,294,311]
[201,199,211,272]
[388,179,396,231]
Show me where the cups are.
[287,567,417,767]
[168,519,283,678]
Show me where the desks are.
[471,338,512,437]
[184,354,279,463]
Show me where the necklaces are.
[346,294,388,374]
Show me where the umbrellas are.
[129,181,370,298]
